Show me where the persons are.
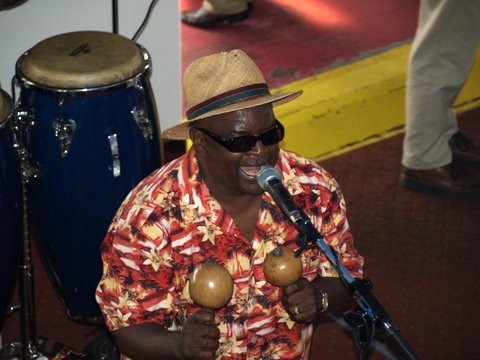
[95,49,364,360]
[180,0,254,28]
[398,0,480,197]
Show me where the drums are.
[1,87,27,324]
[17,28,163,328]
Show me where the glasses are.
[197,119,284,152]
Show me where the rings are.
[294,305,300,315]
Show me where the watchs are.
[311,285,329,315]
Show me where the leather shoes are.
[448,131,480,163]
[406,160,480,195]
[181,2,252,26]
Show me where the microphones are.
[257,166,308,234]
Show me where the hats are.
[161,49,303,140]
